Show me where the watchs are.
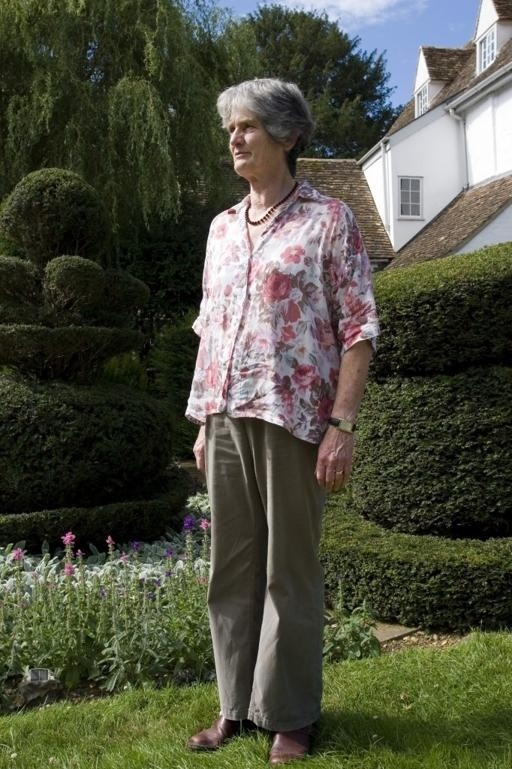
[326,418,357,433]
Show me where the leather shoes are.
[186,715,249,753]
[264,731,311,769]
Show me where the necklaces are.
[245,180,299,228]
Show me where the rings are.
[336,470,344,475]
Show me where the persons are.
[180,76,383,766]
